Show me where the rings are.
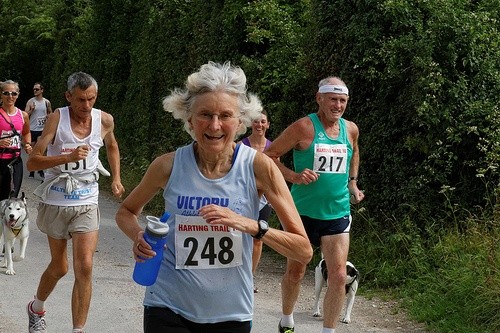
[136,243,140,247]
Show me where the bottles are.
[132,212,171,286]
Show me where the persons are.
[25,82,52,177]
[115,61,313,332]
[0,80,33,253]
[237,109,280,294]
[262,77,364,333]
[26,71,125,333]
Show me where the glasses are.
[1,91,18,96]
[33,88,40,91]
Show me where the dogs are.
[0,190,30,275]
[312,250,360,325]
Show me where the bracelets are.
[25,142,31,146]
[349,177,357,181]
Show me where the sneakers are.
[278,317,294,333]
[72,328,85,333]
[26,295,48,333]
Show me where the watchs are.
[251,220,269,239]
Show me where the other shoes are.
[29,172,34,177]
[38,171,44,177]
